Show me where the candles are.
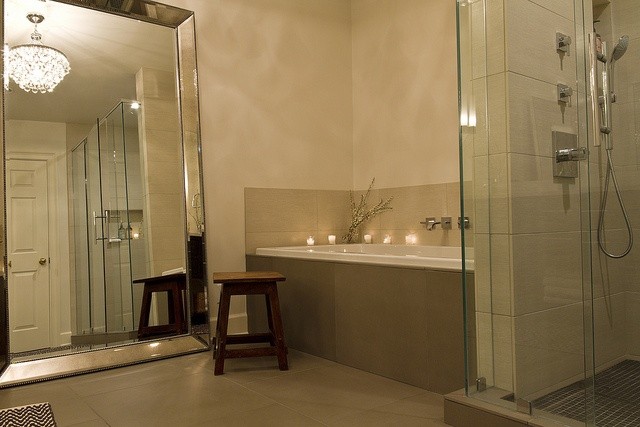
[384,237,391,244]
[364,235,372,243]
[328,235,336,244]
[406,235,417,244]
[133,233,139,239]
[307,238,314,245]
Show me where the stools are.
[133,273,188,339]
[213,272,289,375]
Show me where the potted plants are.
[342,177,394,242]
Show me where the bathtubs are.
[279,243,475,262]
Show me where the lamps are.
[8,14,72,93]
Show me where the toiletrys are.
[128,220,134,239]
[162,267,186,277]
[119,220,126,240]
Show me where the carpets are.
[0,401,57,427]
